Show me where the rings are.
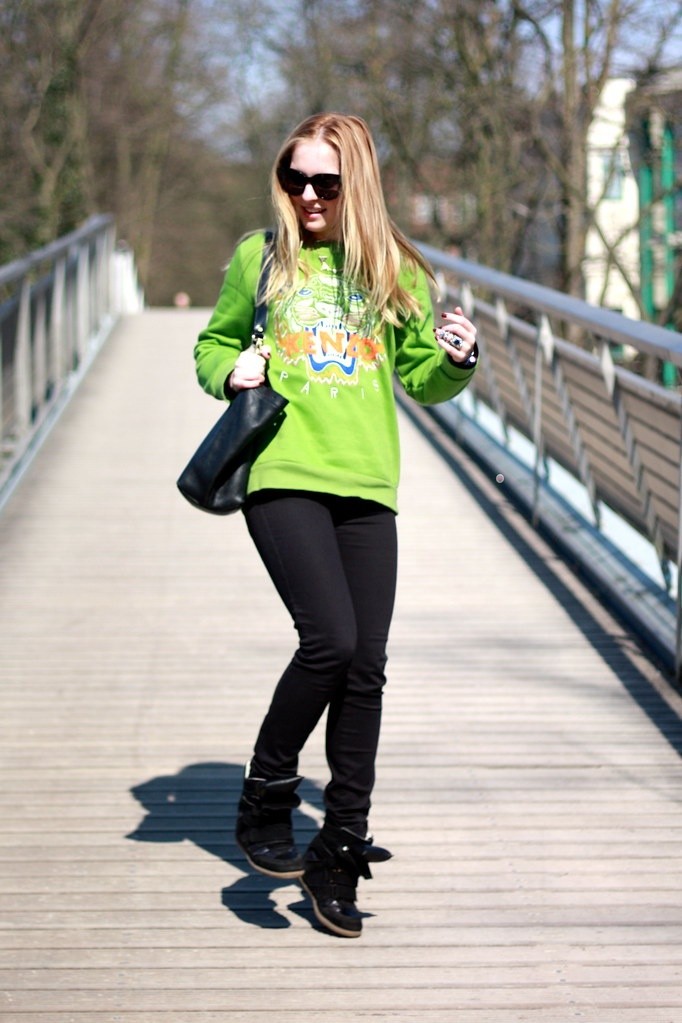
[438,330,464,350]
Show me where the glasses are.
[277,167,341,200]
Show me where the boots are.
[297,819,393,938]
[236,754,305,879]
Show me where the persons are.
[195,113,480,937]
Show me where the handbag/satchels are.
[177,230,290,514]
